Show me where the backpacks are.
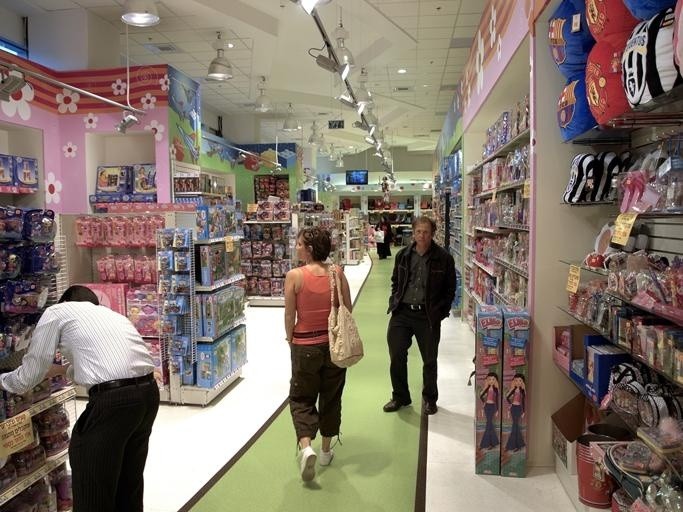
[374,230,384,243]
[36,248,62,272]
[24,208,57,241]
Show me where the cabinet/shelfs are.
[241,203,295,307]
[63,70,201,407]
[366,195,417,230]
[341,211,365,266]
[295,211,336,265]
[432,159,448,250]
[1,49,64,512]
[462,0,615,469]
[445,136,464,317]
[152,175,245,408]
[418,194,435,221]
[554,0,682,512]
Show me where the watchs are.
[285,337,292,342]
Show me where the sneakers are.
[301,447,317,481]
[319,450,334,465]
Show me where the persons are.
[381,176,389,192]
[383,216,458,416]
[504,374,526,454]
[478,372,499,451]
[375,216,393,259]
[283,227,353,484]
[0,285,160,512]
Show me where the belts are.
[88,372,154,395]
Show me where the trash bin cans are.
[587,423,635,442]
[576,433,617,509]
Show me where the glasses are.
[414,230,431,235]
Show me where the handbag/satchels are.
[374,227,385,243]
[328,264,363,368]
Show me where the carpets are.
[178,239,430,511]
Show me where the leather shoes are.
[383,399,412,412]
[423,400,438,415]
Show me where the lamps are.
[331,6,355,68]
[204,30,233,80]
[282,102,302,131]
[253,76,274,113]
[308,41,350,81]
[319,135,331,157]
[309,120,323,146]
[121,0,159,28]
[329,141,338,161]
[355,65,372,102]
[336,149,344,168]
[365,103,378,127]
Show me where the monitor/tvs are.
[345,169,369,185]
